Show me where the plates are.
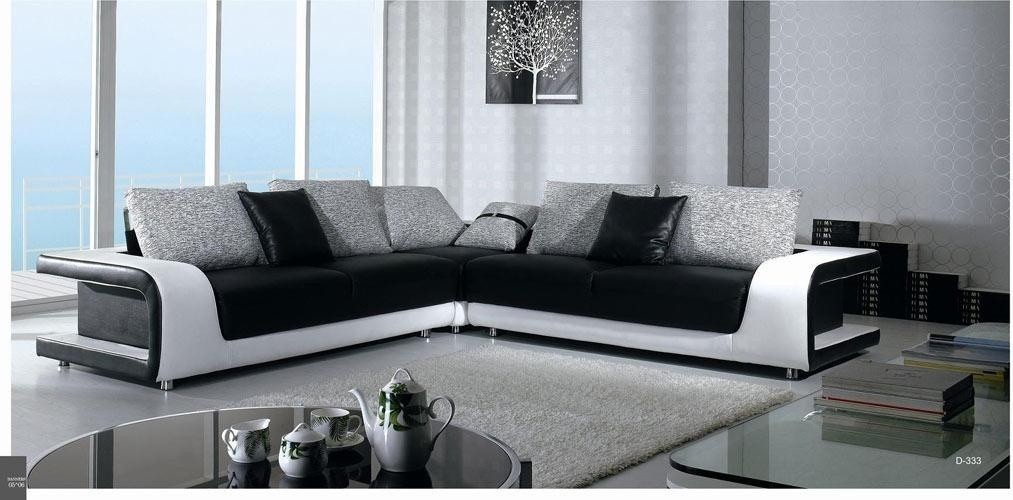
[314,432,365,450]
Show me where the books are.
[811,218,1009,424]
[821,408,973,457]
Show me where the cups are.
[279,424,330,481]
[302,406,362,442]
[221,416,273,463]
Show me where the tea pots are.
[349,365,456,475]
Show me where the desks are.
[18,401,533,488]
